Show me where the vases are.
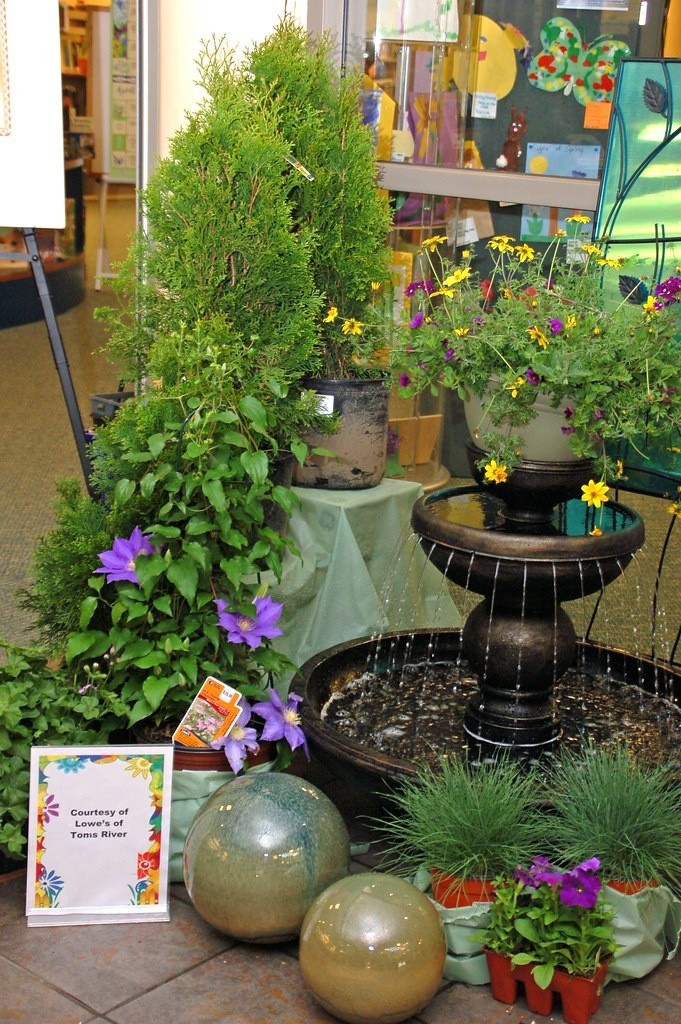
[463,374,600,462]
[481,944,614,1024]
[129,716,278,772]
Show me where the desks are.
[242,478,463,704]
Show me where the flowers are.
[468,856,626,989]
[322,215,681,536]
[63,340,311,775]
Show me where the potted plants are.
[530,724,681,954]
[191,0,397,491]
[355,734,548,909]
